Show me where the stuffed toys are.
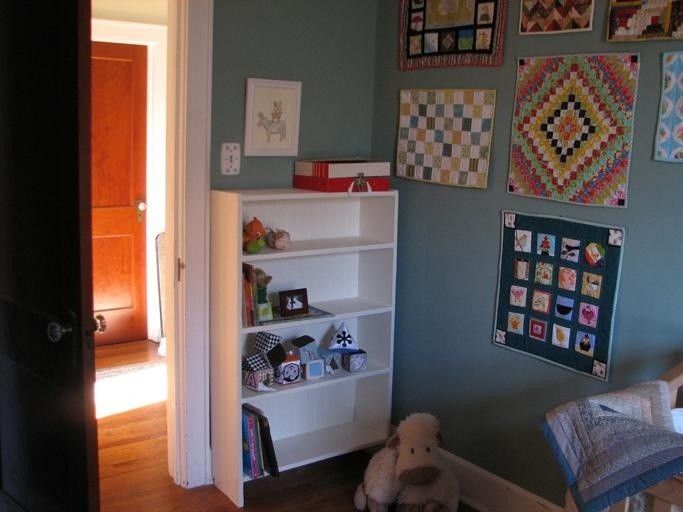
[243,216,291,253]
[355,413,460,512]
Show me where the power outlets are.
[221,142,241,175]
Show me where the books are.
[242,263,258,327]
[242,402,280,479]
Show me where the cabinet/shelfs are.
[209,187,399,511]
[243,75,302,158]
